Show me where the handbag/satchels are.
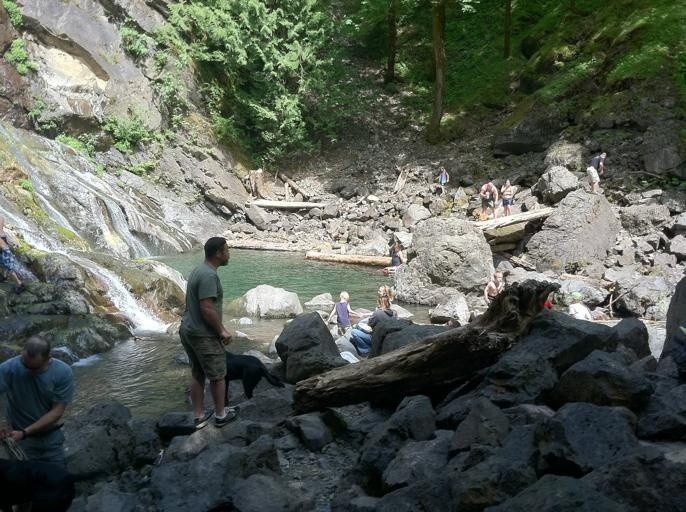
[357,323,372,334]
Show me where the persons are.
[543,298,554,310]
[476,180,501,219]
[498,178,516,216]
[178,237,241,430]
[434,165,450,189]
[327,290,364,337]
[585,153,607,197]
[0,334,75,468]
[566,290,594,323]
[483,270,505,306]
[448,318,461,330]
[350,283,397,358]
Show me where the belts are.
[28,423,64,436]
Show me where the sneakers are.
[215,406,240,428]
[194,409,214,429]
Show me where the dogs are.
[224,350,286,407]
